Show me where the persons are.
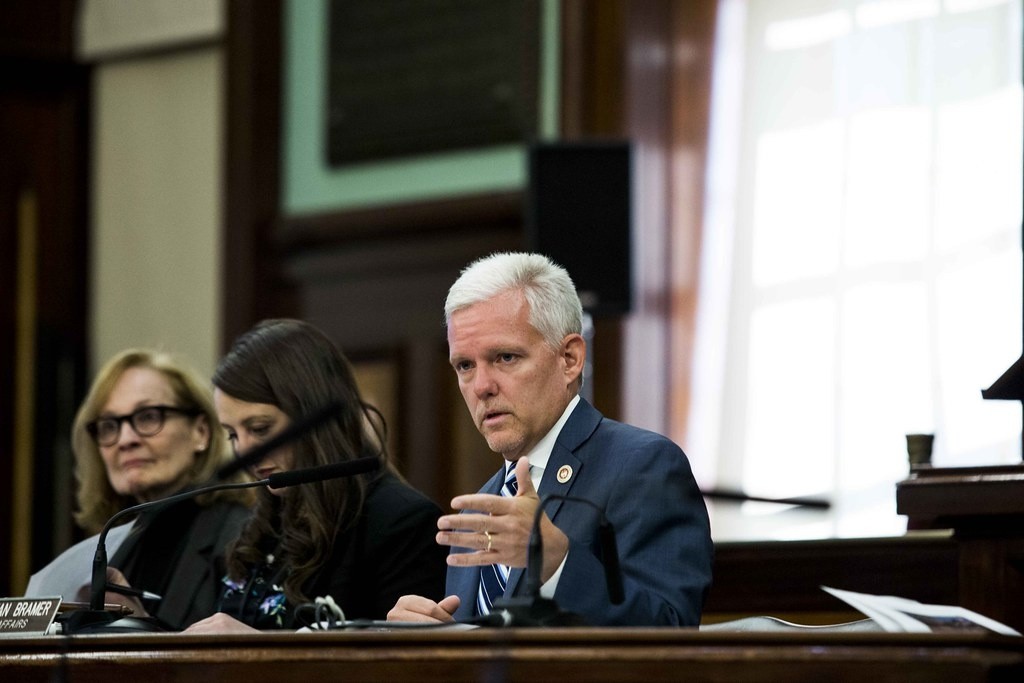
[387,254,714,627]
[79,319,453,634]
[74,348,256,629]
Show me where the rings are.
[481,532,492,553]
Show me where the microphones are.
[490,493,626,629]
[57,454,384,635]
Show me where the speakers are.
[524,139,631,320]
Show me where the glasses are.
[85,405,198,447]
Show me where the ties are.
[474,461,533,616]
